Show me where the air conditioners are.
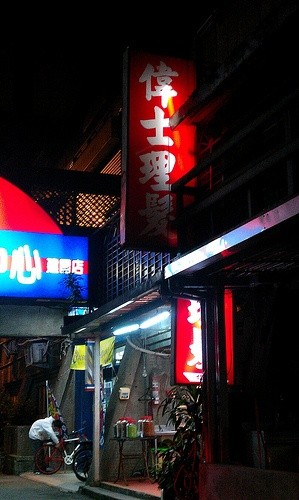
[26,342,48,367]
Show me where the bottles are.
[114,414,155,439]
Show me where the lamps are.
[141,340,148,378]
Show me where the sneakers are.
[45,467,52,471]
[35,472,40,475]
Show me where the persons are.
[28,416,63,475]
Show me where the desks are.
[109,435,154,485]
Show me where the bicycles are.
[35,423,93,474]
[72,438,93,482]
